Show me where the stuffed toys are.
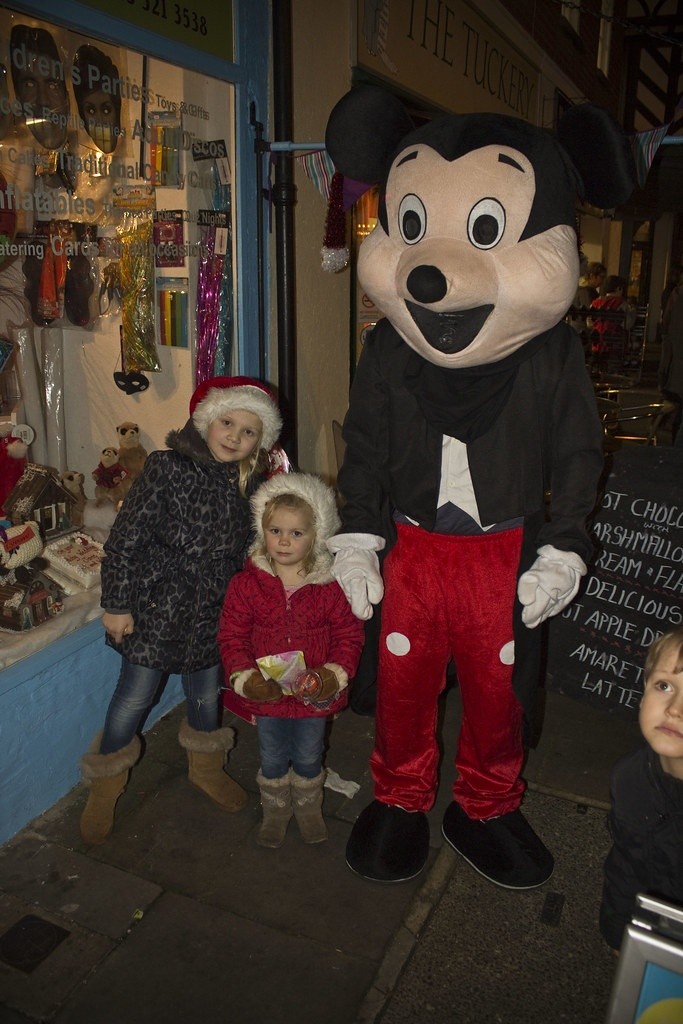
[0,435,29,519]
[116,422,149,486]
[91,448,127,511]
[321,77,629,891]
[59,472,89,528]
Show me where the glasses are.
[595,274,607,279]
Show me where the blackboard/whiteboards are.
[524,452,682,807]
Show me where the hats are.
[191,376,283,451]
[249,473,342,556]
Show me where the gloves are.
[299,663,347,706]
[233,669,283,702]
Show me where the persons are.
[597,631,683,954]
[217,474,365,850]
[78,376,284,846]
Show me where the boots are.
[79,734,142,839]
[256,766,328,847]
[179,717,251,813]
